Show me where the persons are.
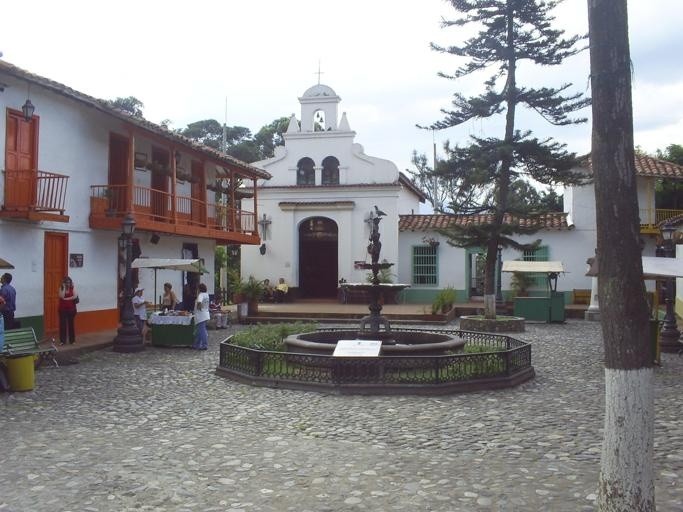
[208,295,229,330]
[271,277,288,303]
[0,272,16,330]
[259,278,273,303]
[0,297,11,392]
[56,276,77,346]
[129,286,149,345]
[191,283,211,350]
[160,281,176,311]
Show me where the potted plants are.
[225,270,268,313]
[422,285,456,321]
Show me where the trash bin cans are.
[7,353,34,391]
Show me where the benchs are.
[1,325,60,372]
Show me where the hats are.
[134,287,144,293]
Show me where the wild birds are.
[374,206,387,217]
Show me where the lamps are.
[20,82,34,121]
